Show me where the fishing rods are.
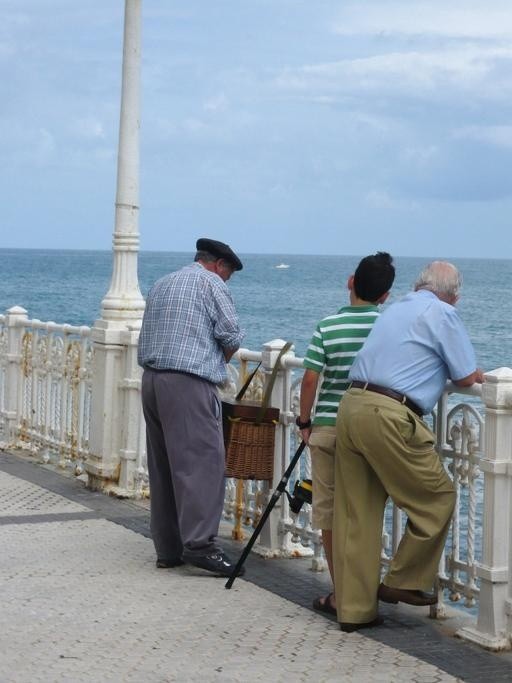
[225,438,312,588]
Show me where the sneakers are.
[157,553,244,577]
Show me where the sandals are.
[314,592,338,614]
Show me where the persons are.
[332,260,483,632]
[300,253,396,615]
[137,238,245,577]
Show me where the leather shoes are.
[377,583,436,605]
[349,379,422,416]
[341,617,384,634]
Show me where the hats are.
[196,239,243,273]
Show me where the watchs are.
[296,416,311,430]
